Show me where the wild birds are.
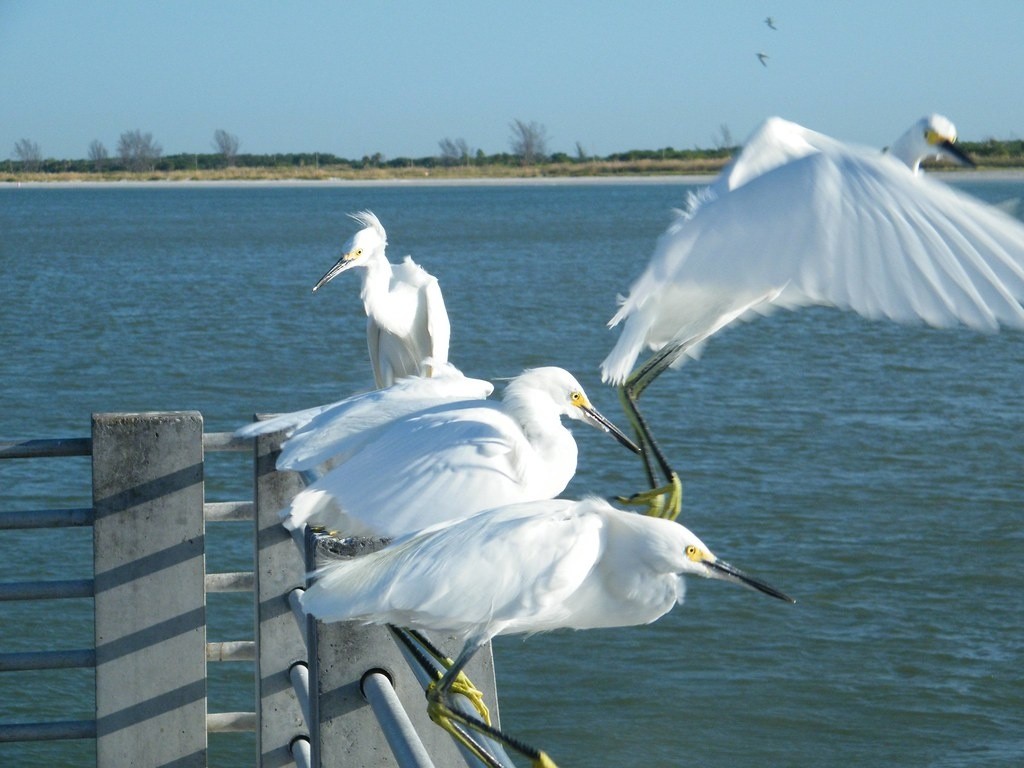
[312,205,452,388]
[299,498,798,768]
[278,364,646,538]
[599,110,1023,520]
[230,360,494,473]
